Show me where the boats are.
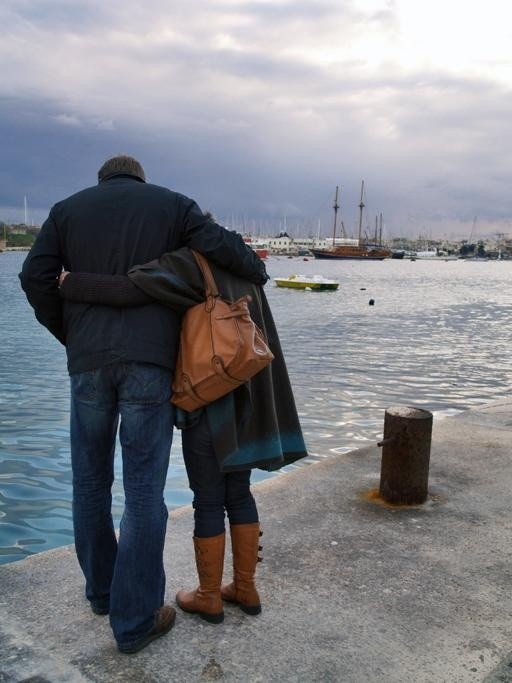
[273,274,339,290]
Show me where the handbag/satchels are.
[169,294,275,414]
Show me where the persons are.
[53,205,311,622]
[20,152,271,653]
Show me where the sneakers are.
[119,606,175,654]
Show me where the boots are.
[221,522,262,615]
[176,529,224,624]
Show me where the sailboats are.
[242,179,406,260]
